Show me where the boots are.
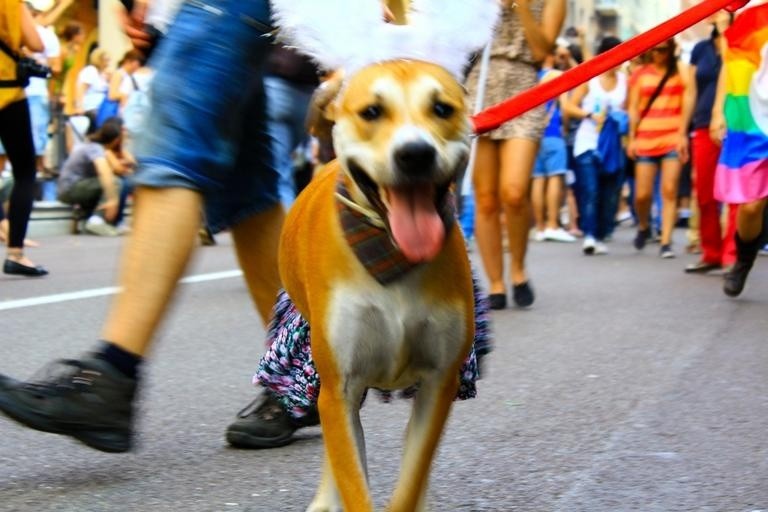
[724,229,761,296]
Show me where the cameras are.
[17,58,51,86]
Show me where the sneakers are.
[660,245,674,259]
[198,223,215,245]
[526,226,613,256]
[514,283,532,306]
[226,387,319,449]
[86,219,117,238]
[487,293,505,308]
[0,356,136,453]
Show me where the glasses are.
[652,45,668,53]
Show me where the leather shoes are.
[684,261,722,273]
[4,259,48,276]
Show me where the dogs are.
[275,58,477,512]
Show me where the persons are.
[459,1,766,295]
[1,0,321,455]
[464,0,569,311]
[185,20,336,249]
[1,1,178,280]
